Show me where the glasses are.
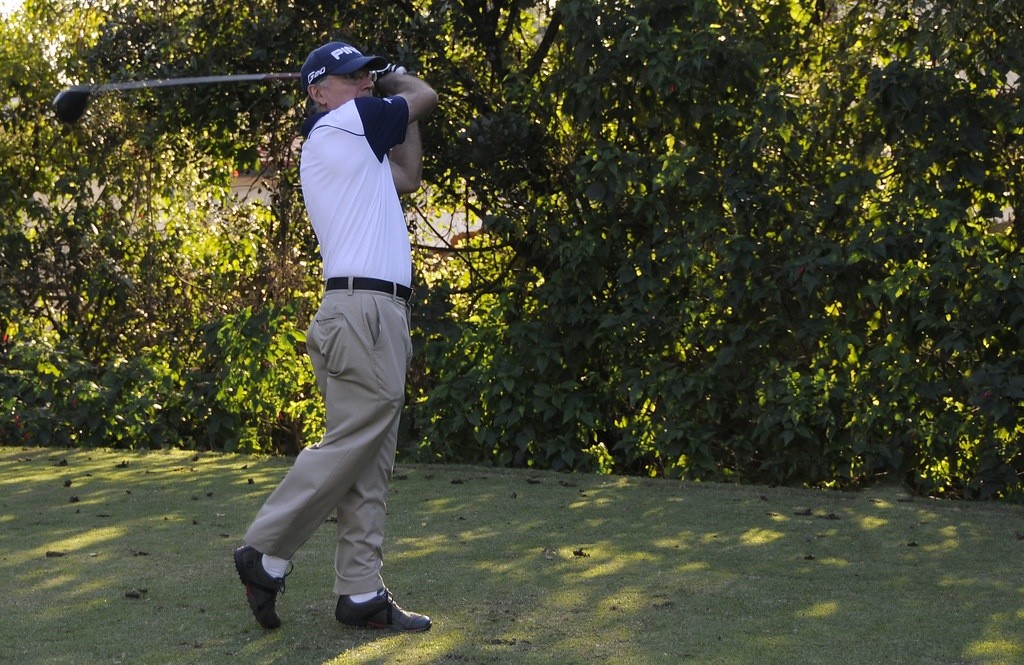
[324,69,377,86]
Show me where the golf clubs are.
[49,65,301,126]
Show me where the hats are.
[301,42,387,97]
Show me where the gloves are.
[387,63,407,75]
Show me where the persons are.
[234,41,439,633]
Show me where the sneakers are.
[233,545,293,630]
[335,588,432,633]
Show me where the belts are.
[325,276,414,303]
